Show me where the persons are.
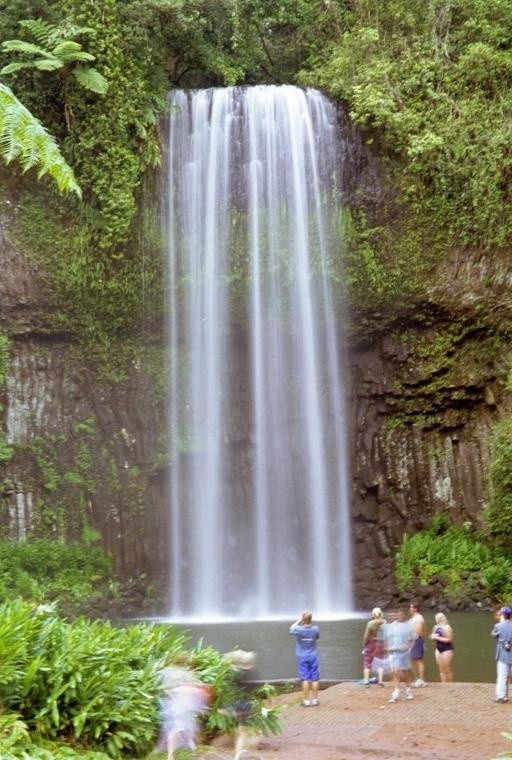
[406,604,427,688]
[491,607,512,703]
[362,606,386,687]
[289,612,320,707]
[387,608,415,702]
[430,612,455,682]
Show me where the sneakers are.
[405,686,414,700]
[388,688,400,703]
[413,679,426,688]
[358,679,370,685]
[377,682,384,688]
[311,699,320,706]
[300,700,311,707]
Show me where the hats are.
[504,608,512,614]
